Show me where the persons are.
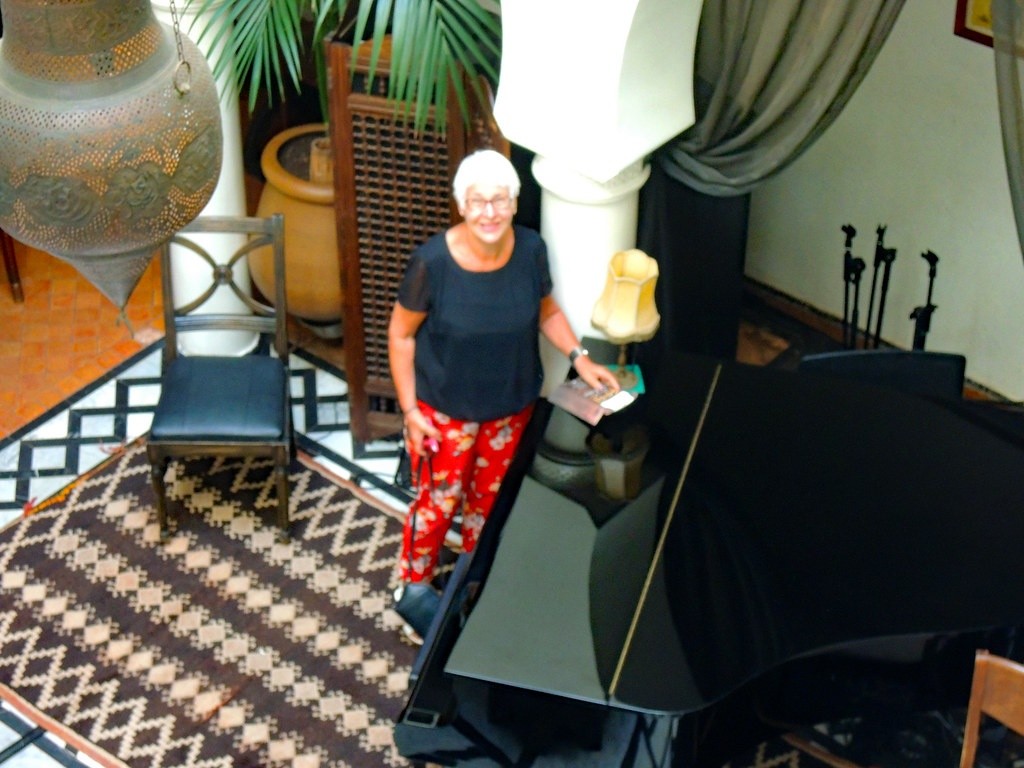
[388,150,618,644]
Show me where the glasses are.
[467,193,512,209]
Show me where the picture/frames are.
[953,0,1024,57]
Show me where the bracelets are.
[403,405,418,415]
[569,346,588,363]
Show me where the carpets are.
[0,433,462,768]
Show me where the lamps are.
[0,0,223,337]
[592,249,662,364]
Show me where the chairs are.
[145,213,292,544]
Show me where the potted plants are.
[179,1,500,317]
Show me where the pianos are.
[393,347,1024,767]
